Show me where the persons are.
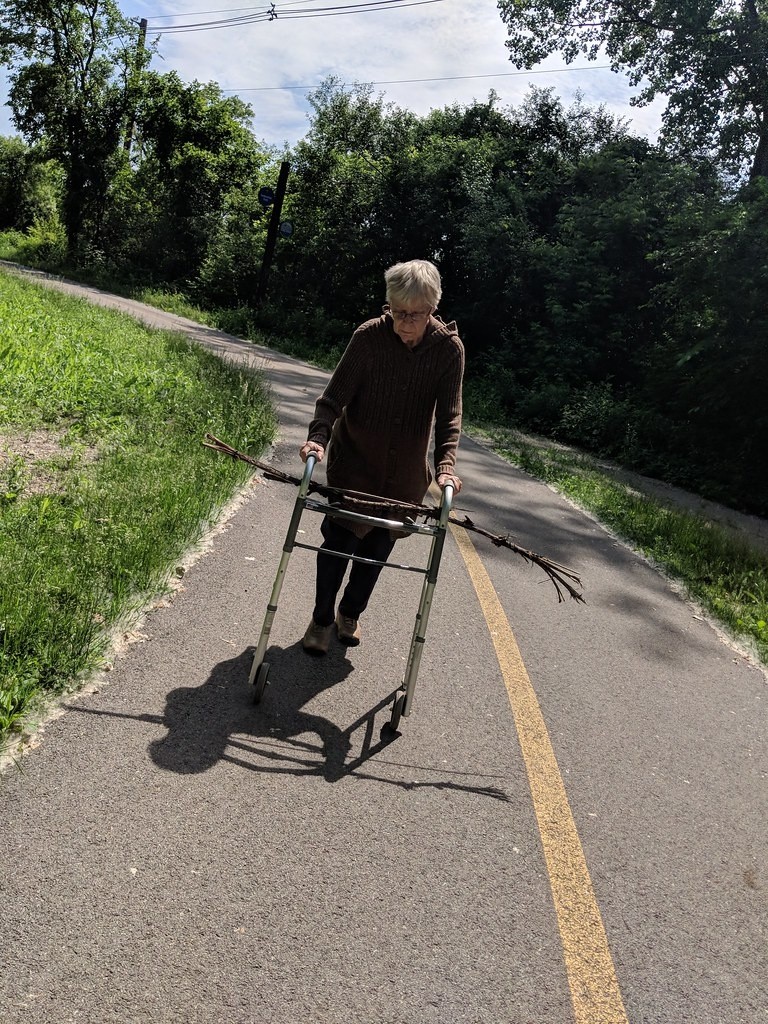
[299,260,465,657]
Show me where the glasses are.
[389,305,433,321]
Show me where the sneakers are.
[304,618,335,658]
[336,609,358,639]
